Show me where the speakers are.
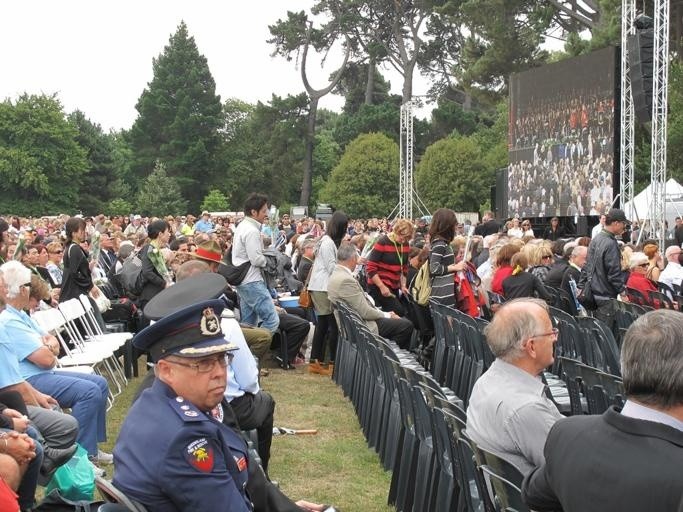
[577,217,588,236]
[402,132,415,171]
[491,186,496,210]
[628,32,670,121]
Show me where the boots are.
[38,440,77,476]
[308,359,334,374]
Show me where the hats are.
[609,209,632,225]
[189,241,228,265]
[133,273,240,362]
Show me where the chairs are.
[1,279,136,502]
[249,280,682,512]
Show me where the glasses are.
[166,353,234,373]
[638,263,649,267]
[532,328,558,341]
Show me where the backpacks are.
[412,259,431,306]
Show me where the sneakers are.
[90,451,114,477]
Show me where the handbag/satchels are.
[578,281,597,311]
[88,284,113,314]
[298,291,313,308]
[218,245,251,285]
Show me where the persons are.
[0,195,325,371]
[464,296,570,478]
[0,258,327,511]
[521,309,682,510]
[307,208,683,364]
[507,75,612,218]
